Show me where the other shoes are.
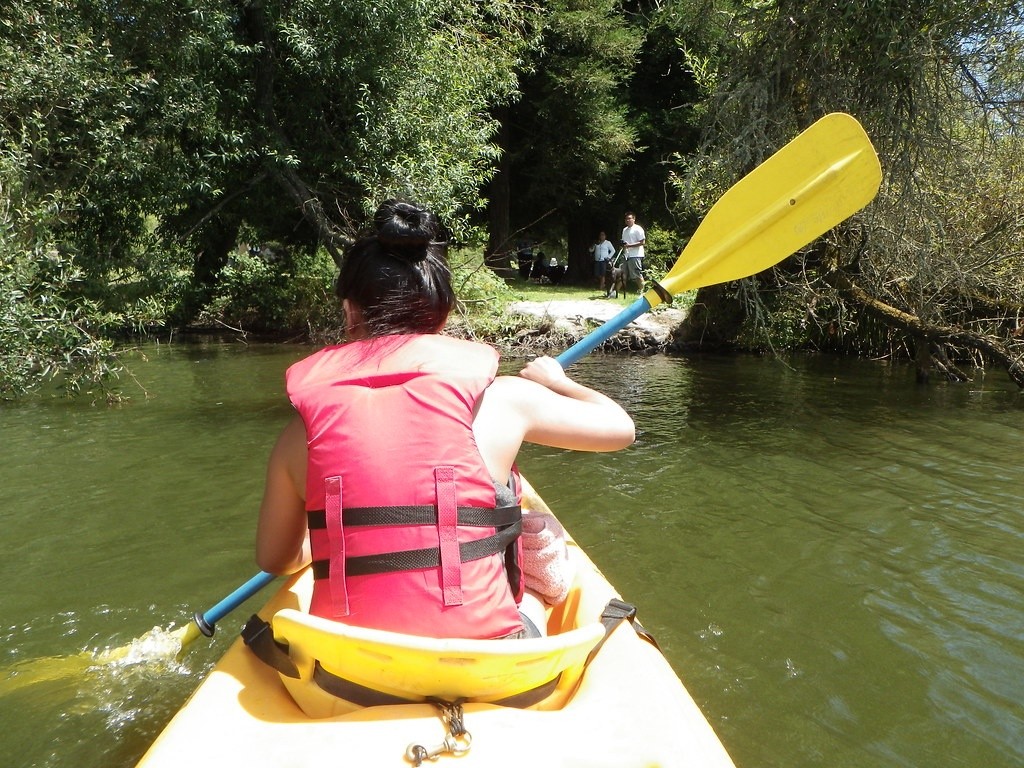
[636,290,643,299]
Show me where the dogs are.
[604,257,627,299]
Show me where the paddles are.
[167,112,885,648]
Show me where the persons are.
[620,212,645,294]
[531,251,552,279]
[590,231,617,289]
[256,200,637,707]
[514,229,532,280]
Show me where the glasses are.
[625,217,633,220]
